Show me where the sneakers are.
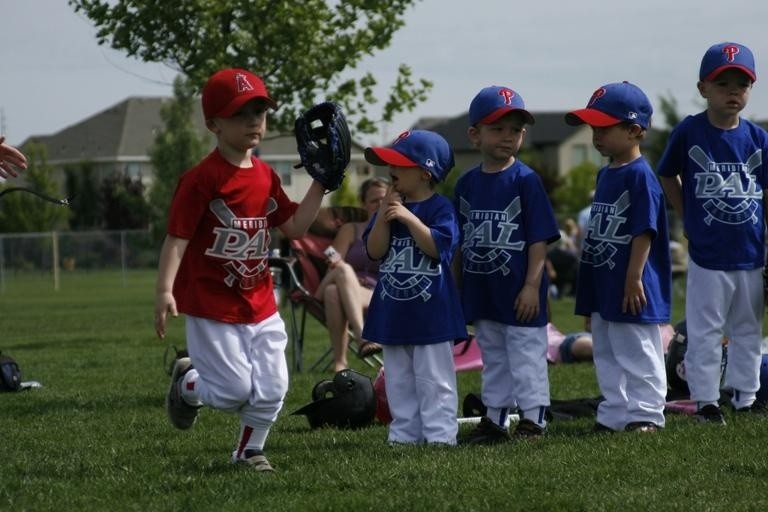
[730,399,766,416]
[575,422,616,436]
[694,405,725,426]
[231,448,274,473]
[471,416,511,445]
[515,418,546,436]
[167,356,199,431]
[622,422,659,435]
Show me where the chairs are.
[263,254,306,374]
[547,248,579,299]
[288,204,386,375]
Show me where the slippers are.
[357,340,384,359]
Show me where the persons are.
[547,299,593,363]
[316,177,390,373]
[0,136,28,179]
[154,67,351,471]
[546,218,581,297]
[578,191,596,251]
[654,42,768,428]
[361,130,469,447]
[564,81,671,432]
[453,85,561,442]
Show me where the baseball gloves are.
[293,102,351,194]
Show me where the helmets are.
[372,366,394,425]
[290,370,374,431]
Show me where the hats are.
[565,80,653,132]
[468,86,535,127]
[202,68,278,120]
[364,129,455,184]
[700,43,757,82]
